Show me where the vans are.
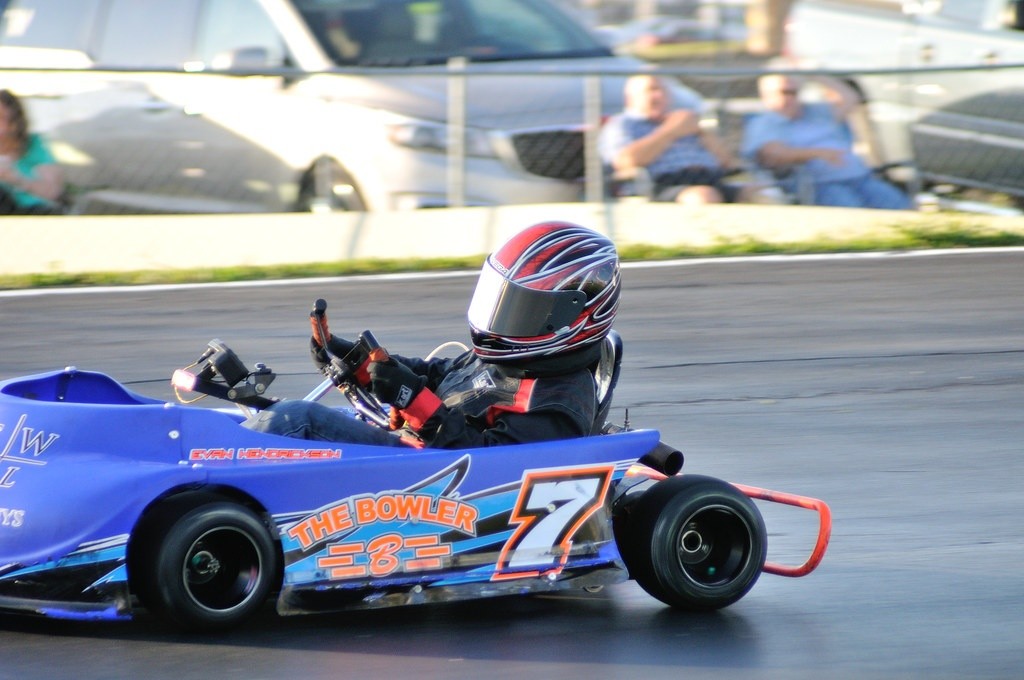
[0,0,1024,214]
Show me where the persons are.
[741,74,919,210]
[240,219,621,452]
[0,90,65,216]
[601,74,770,206]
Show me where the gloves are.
[310,335,355,370]
[367,356,426,410]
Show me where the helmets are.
[467,221,622,363]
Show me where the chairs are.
[593,330,623,434]
